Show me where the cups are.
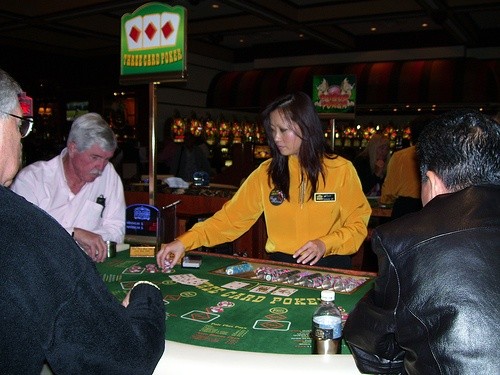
[104,241,117,257]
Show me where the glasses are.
[4,111,34,138]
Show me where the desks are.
[125,185,393,259]
[40,243,379,375]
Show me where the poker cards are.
[168,273,211,287]
[249,284,299,297]
[221,278,249,290]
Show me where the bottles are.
[313,290,342,354]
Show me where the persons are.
[342,109,500,375]
[367,115,423,221]
[10,112,126,263]
[156,91,372,270]
[0,69,166,375]
